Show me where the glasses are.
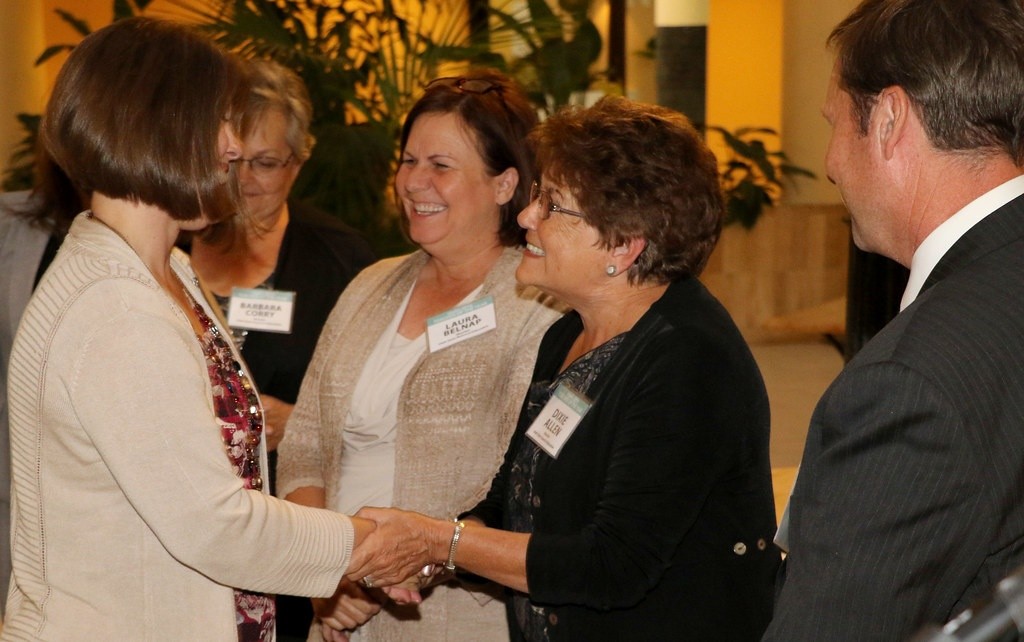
[228,152,293,179]
[425,77,509,119]
[529,179,583,221]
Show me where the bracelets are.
[444,522,465,573]
[448,515,458,524]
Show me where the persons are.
[761,0,1024,642]
[343,94,777,642]
[175,62,341,642]
[0,16,436,642]
[273,75,571,642]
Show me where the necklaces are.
[87,211,263,491]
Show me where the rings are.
[421,564,431,576]
[364,577,373,588]
